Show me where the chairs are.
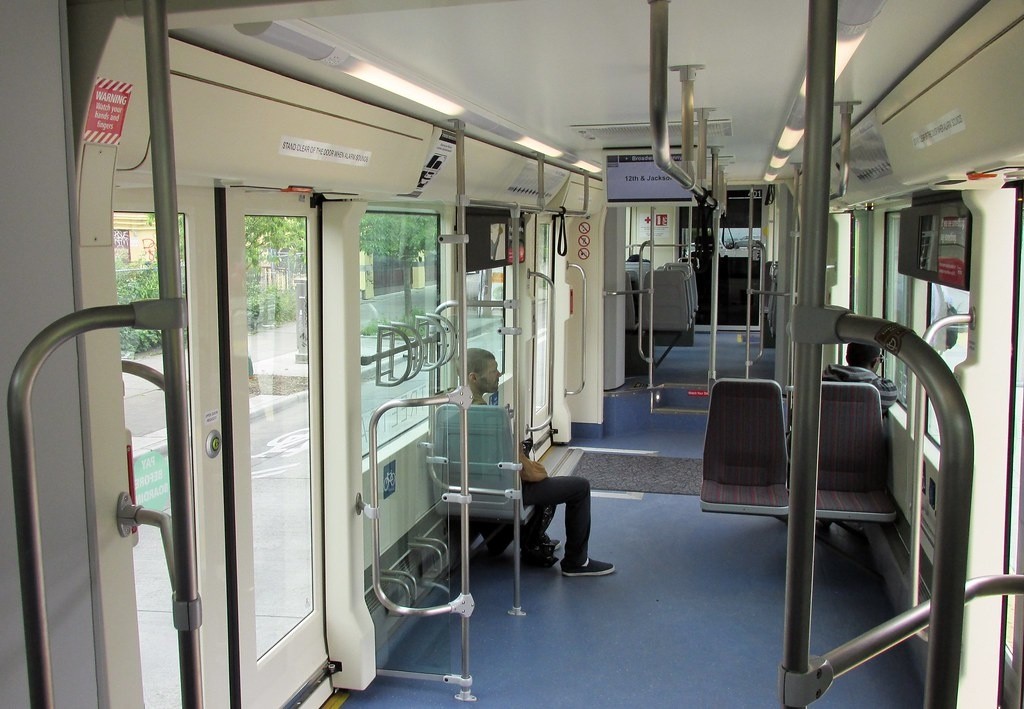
[698,377,888,583]
[819,381,898,543]
[625,259,701,368]
[428,403,537,571]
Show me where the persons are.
[435,347,615,576]
[784,342,900,527]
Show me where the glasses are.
[873,354,884,363]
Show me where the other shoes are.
[525,540,561,554]
[816,519,832,535]
[562,558,615,577]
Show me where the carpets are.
[568,451,703,496]
